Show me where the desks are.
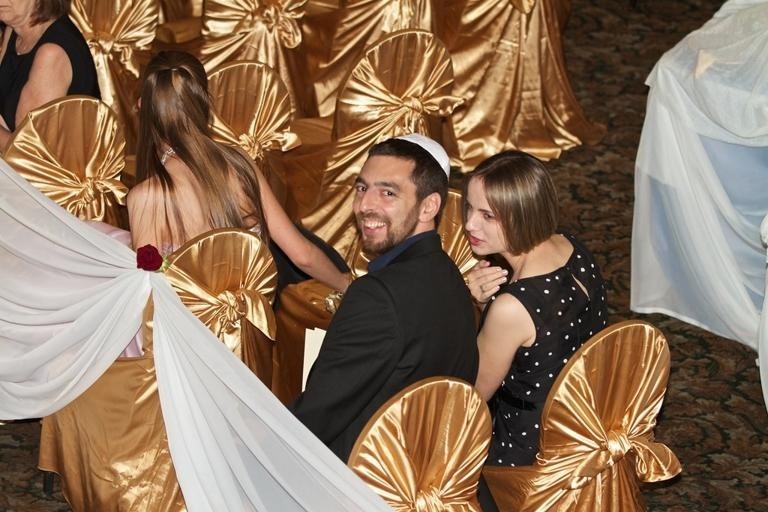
[633,1,767,350]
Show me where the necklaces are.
[14,26,46,55]
[159,145,177,166]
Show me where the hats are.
[395,131,452,179]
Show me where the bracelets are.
[340,272,354,295]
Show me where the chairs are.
[483,319,682,510]
[282,188,492,401]
[38,230,281,511]
[346,377,493,512]
[205,62,295,165]
[428,2,586,171]
[284,29,458,272]
[317,1,436,121]
[68,1,171,157]
[3,94,131,230]
[201,0,317,120]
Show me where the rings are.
[479,285,485,293]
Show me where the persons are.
[287,132,480,466]
[0,0,101,162]
[458,149,607,512]
[123,50,354,296]
